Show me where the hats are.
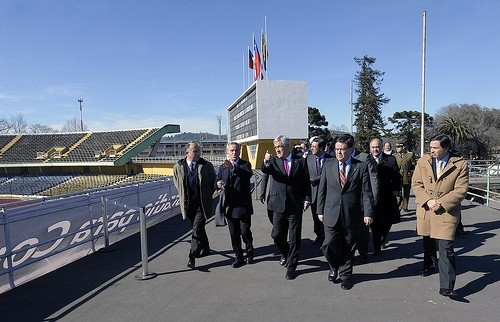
[394,141,405,148]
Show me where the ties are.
[283,159,290,176]
[316,157,322,175]
[230,164,235,177]
[339,162,347,189]
[435,160,443,182]
[190,163,194,173]
[400,153,402,158]
[375,157,380,164]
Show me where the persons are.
[316,134,374,290]
[294,136,401,263]
[214,142,254,269]
[261,135,311,280]
[172,141,217,267]
[392,140,417,212]
[411,134,470,296]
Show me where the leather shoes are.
[246,251,253,264]
[341,279,350,290]
[419,266,436,276]
[232,259,244,268]
[188,258,195,268]
[286,267,295,280]
[328,268,338,281]
[280,255,288,266]
[439,288,454,296]
[197,249,209,258]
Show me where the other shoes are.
[272,245,280,255]
[403,208,410,212]
[374,250,380,255]
[361,254,372,263]
[312,237,321,247]
[381,243,386,249]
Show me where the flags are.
[253,41,261,81]
[248,50,263,79]
[262,36,269,71]
